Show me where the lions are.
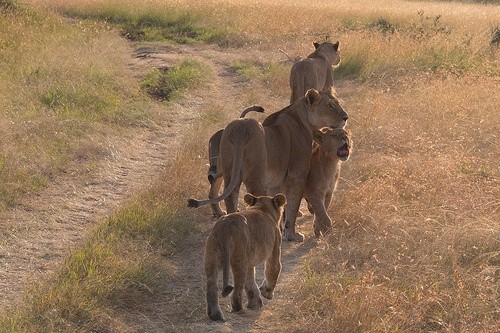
[204,194,286,323]
[187,89,350,242]
[206,128,316,217]
[289,41,341,104]
[240,105,354,236]
